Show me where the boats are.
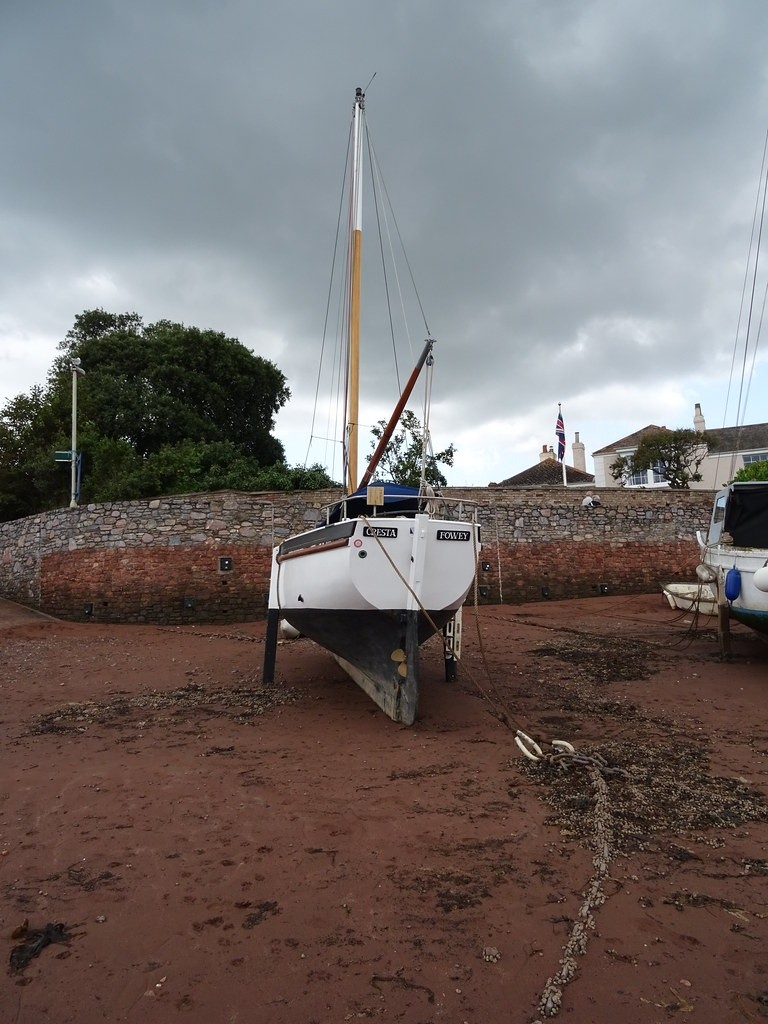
[657,581,720,618]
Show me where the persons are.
[556,414,565,460]
[582,491,600,505]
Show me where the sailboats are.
[261,72,481,683]
[693,130,768,660]
